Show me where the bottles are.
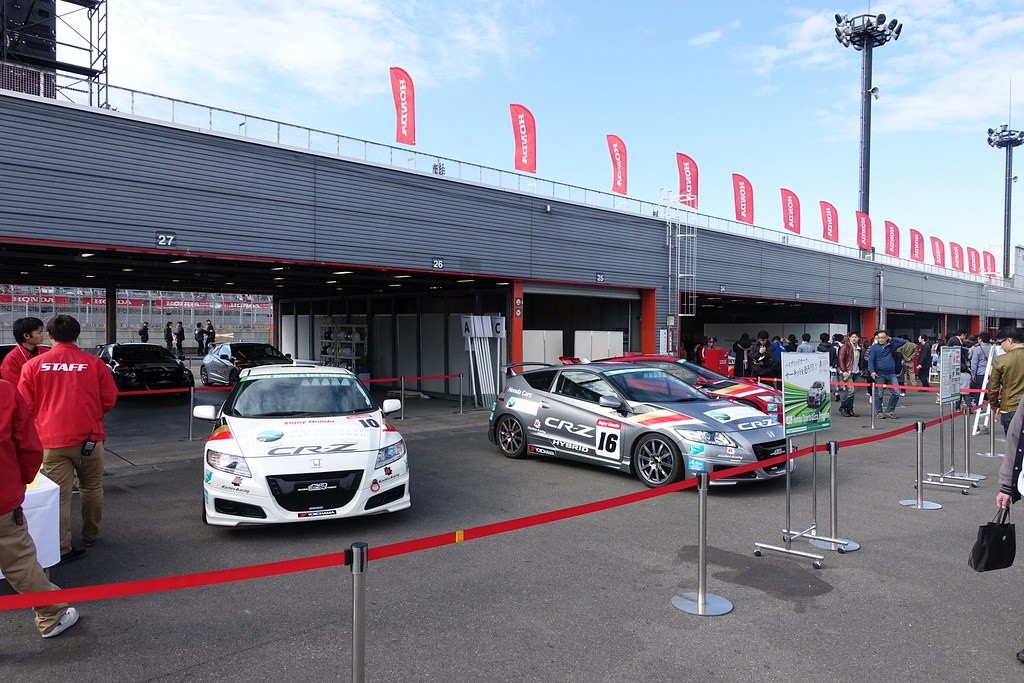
[842,371,851,382]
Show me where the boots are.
[838,404,850,417]
[847,406,860,417]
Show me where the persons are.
[1,316,81,494]
[680,330,1024,419]
[195,320,216,356]
[139,321,149,343]
[164,322,185,355]
[987,326,1024,509]
[2,377,79,638]
[17,315,119,564]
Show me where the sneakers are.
[58,548,86,563]
[876,412,885,419]
[885,408,897,419]
[41,605,79,638]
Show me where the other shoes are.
[835,395,840,402]
[900,393,905,397]
[870,396,872,403]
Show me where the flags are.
[930,237,945,267]
[510,103,536,174]
[676,152,698,209]
[820,201,838,242]
[910,229,924,263]
[856,211,872,250]
[607,134,627,195]
[732,173,754,224]
[389,67,416,146]
[885,221,900,257]
[781,188,800,234]
[950,242,996,277]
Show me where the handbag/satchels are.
[968,505,1016,572]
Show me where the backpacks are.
[895,352,906,376]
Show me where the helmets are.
[706,337,714,342]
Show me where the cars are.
[200,342,291,386]
[559,351,783,426]
[192,364,412,528]
[806,381,826,408]
[99,340,196,392]
[488,356,797,489]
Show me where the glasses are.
[998,338,1007,345]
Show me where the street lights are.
[986,77,1024,277]
[834,1,903,220]
